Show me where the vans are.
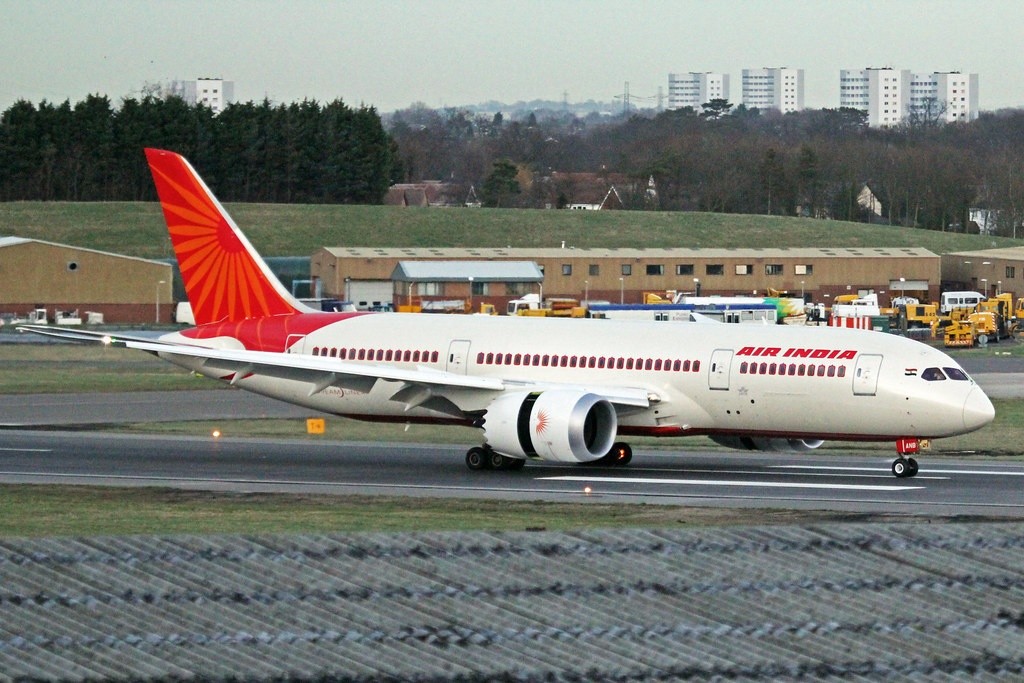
[940,290,987,313]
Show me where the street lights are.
[693,278,698,296]
[155,280,167,324]
[584,280,587,306]
[801,281,804,297]
[981,278,988,297]
[900,278,905,304]
[619,277,624,304]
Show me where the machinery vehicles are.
[392,290,1024,348]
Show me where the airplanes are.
[13,148,995,478]
